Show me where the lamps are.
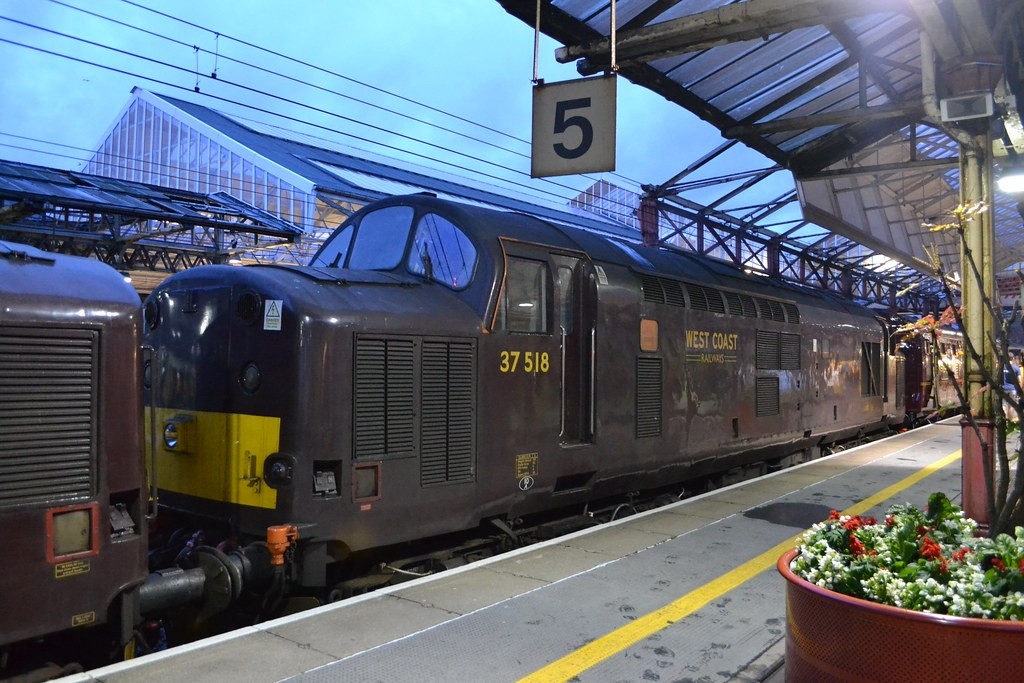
[997,163,1024,193]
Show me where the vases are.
[778,548,1024,683]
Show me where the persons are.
[1012,356,1024,417]
[1002,352,1021,421]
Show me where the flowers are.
[791,492,1024,622]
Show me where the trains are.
[0,186,1014,677]
